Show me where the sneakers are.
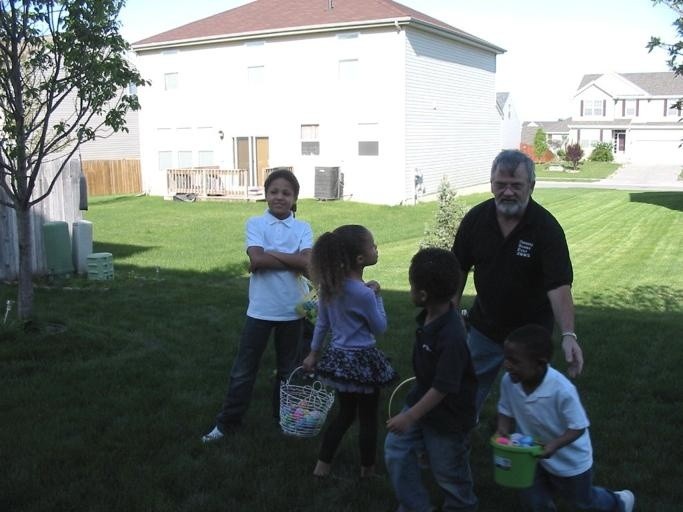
[202,426,223,442]
[614,490,634,512]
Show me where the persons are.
[496,322,636,512]
[384,245,480,512]
[451,147,585,434]
[301,222,398,480]
[202,168,315,443]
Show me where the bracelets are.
[561,330,578,341]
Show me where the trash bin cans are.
[43,221,93,280]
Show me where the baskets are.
[280,366,335,437]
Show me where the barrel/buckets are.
[489,435,547,489]
